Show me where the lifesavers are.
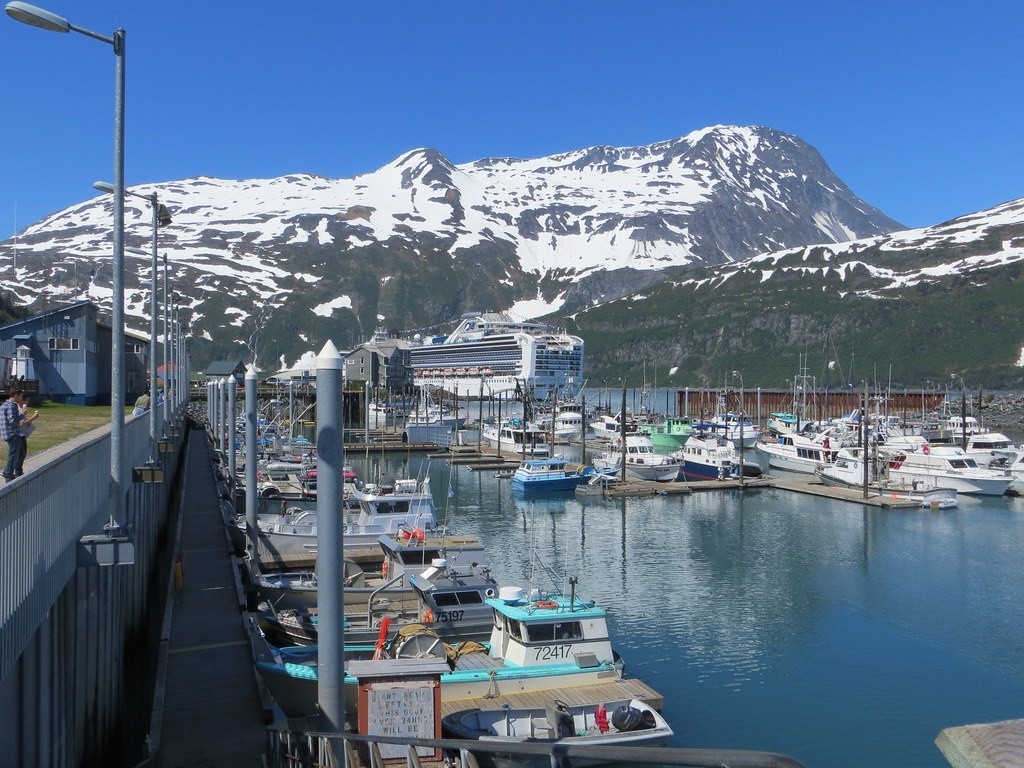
[535,600,560,609]
[923,446,930,455]
[403,526,425,541]
[381,562,388,578]
[421,609,434,623]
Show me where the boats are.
[249,460,489,615]
[754,432,841,475]
[886,446,1016,497]
[440,698,676,768]
[368,397,409,418]
[510,456,622,494]
[265,431,501,647]
[667,421,764,481]
[225,425,363,516]
[225,476,450,557]
[482,418,552,456]
[814,447,960,509]
[405,380,467,431]
[279,498,626,714]
[767,342,990,446]
[534,361,761,449]
[590,430,686,483]
[965,433,1023,496]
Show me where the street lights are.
[140,281,176,422]
[92,180,158,467]
[124,246,170,439]
[158,302,179,411]
[731,370,745,486]
[950,373,966,448]
[4,0,126,525]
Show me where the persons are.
[133,390,151,416]
[158,389,171,404]
[0,386,39,484]
